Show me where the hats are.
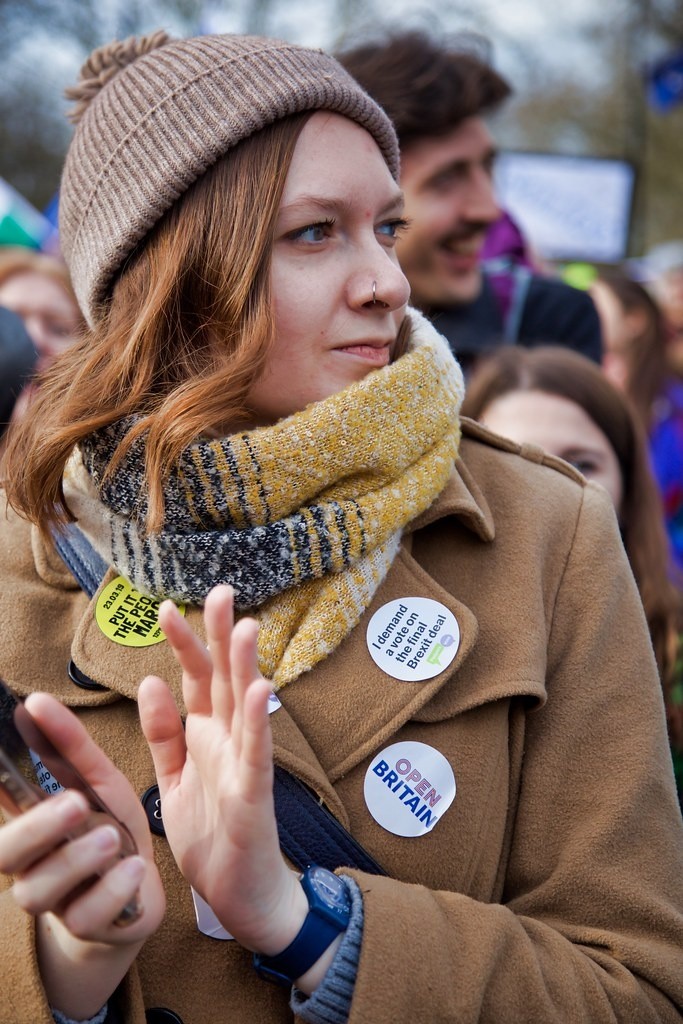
[57,30,402,335]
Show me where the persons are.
[0,31,683,1024]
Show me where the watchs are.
[258,865,352,981]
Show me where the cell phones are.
[1,677,146,928]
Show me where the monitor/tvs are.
[486,148,643,267]
